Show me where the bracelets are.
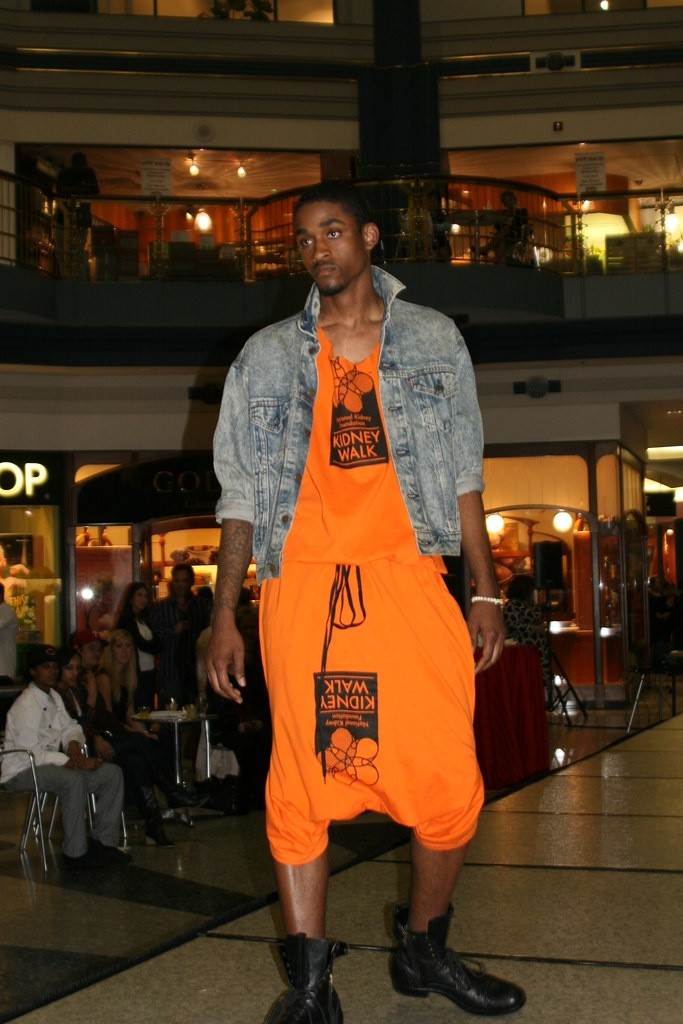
[472,596,505,609]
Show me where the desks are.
[446,209,510,263]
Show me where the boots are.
[260,931,342,1024]
[151,766,209,814]
[135,786,174,849]
[389,902,526,1016]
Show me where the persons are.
[501,573,549,705]
[56,151,99,249]
[208,181,527,1024]
[647,576,683,666]
[56,562,272,843]
[0,581,133,874]
[470,191,527,267]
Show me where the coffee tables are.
[130,709,218,786]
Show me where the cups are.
[138,707,151,719]
[185,704,197,719]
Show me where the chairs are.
[485,226,539,271]
[0,687,127,870]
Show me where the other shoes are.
[62,854,91,878]
[98,845,132,864]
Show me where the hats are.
[72,633,109,649]
[26,645,58,667]
